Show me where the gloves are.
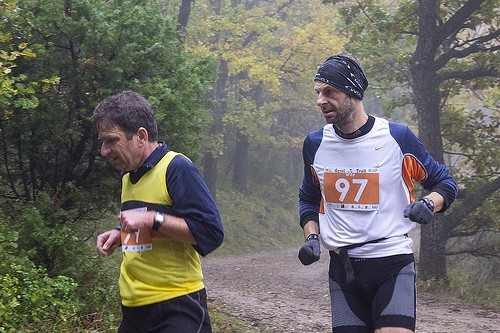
[403,197,436,225]
[298,234,322,266]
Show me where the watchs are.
[419,197,436,213]
[152,211,165,234]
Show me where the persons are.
[298,53,459,333]
[91,90,224,333]
